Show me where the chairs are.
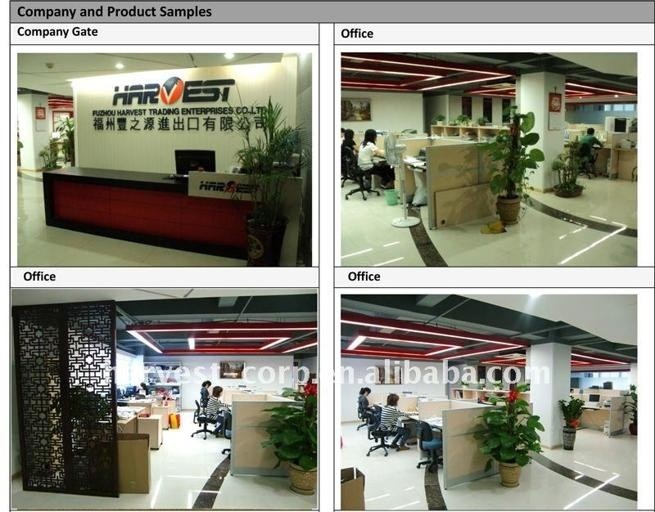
[356,396,442,472]
[341,145,379,200]
[191,400,232,461]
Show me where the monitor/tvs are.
[175,149,215,174]
[589,394,600,402]
[613,117,628,134]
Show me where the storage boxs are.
[341,467,365,511]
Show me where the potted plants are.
[621,384,637,435]
[217,80,312,266]
[473,105,593,225]
[260,383,317,495]
[430,112,471,126]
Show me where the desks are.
[570,388,626,435]
[222,387,305,476]
[569,128,637,181]
[117,394,181,493]
[418,398,506,492]
[354,131,501,229]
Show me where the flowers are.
[558,395,585,450]
[473,384,546,488]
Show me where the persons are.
[380,394,411,450]
[358,129,394,189]
[206,386,229,438]
[358,387,371,413]
[200,381,211,408]
[341,128,359,175]
[570,388,574,395]
[140,382,150,395]
[578,128,603,152]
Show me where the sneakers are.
[212,430,224,437]
[390,444,411,452]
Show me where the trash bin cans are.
[384,190,400,204]
[604,421,610,432]
[170,414,180,428]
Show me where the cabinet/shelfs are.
[451,387,530,403]
[430,125,511,139]
